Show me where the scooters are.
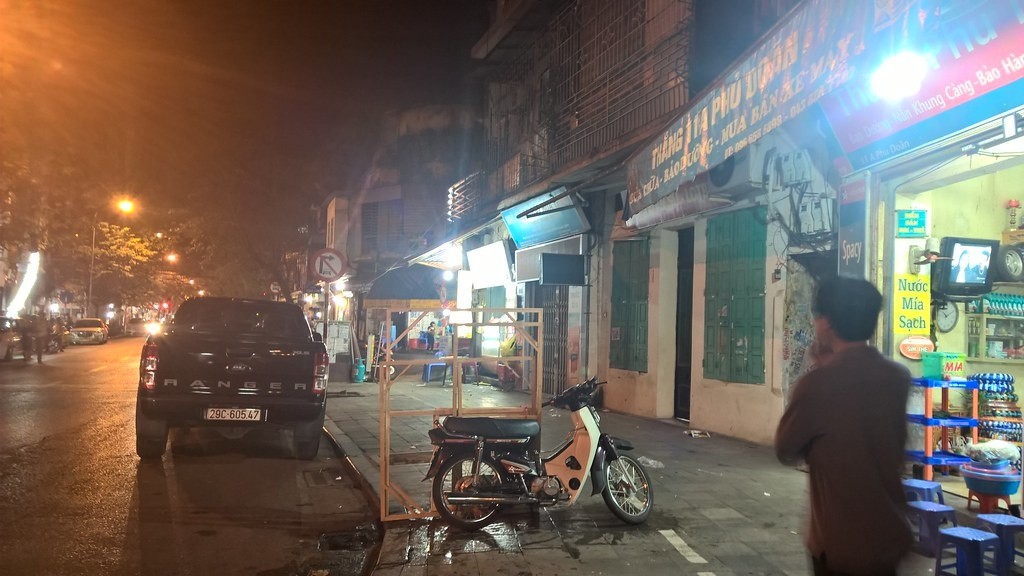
[421,376,656,533]
[359,342,400,379]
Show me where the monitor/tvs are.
[932,237,1000,294]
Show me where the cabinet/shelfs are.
[906,377,979,481]
[964,313,1024,363]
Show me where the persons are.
[775,274,912,576]
[426,322,436,351]
[17,310,74,364]
[950,249,989,284]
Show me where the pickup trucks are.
[134,292,329,459]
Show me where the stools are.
[935,526,1001,576]
[905,500,958,556]
[975,514,1024,576]
[422,363,447,382]
[462,362,480,386]
[967,490,1011,515]
[901,478,948,525]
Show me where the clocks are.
[934,300,959,333]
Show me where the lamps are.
[909,238,943,274]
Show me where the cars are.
[42,323,71,353]
[126,317,162,337]
[69,317,110,344]
[0,315,38,362]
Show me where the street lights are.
[86,197,134,318]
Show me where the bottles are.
[966,292,1024,335]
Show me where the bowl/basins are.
[959,459,1022,496]
